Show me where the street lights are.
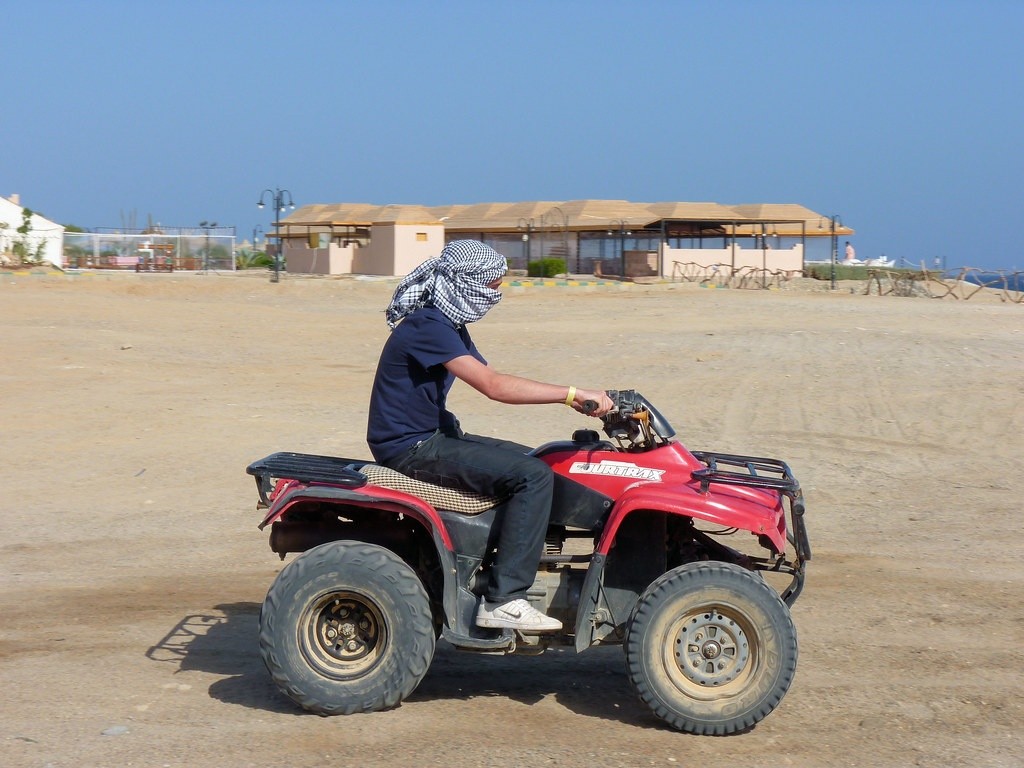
[817,214,844,290]
[253,224,263,251]
[608,219,632,277]
[752,222,777,288]
[257,186,296,283]
[516,217,534,270]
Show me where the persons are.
[845,241,855,259]
[366,238,613,630]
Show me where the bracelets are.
[565,386,576,407]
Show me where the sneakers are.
[475,595,563,630]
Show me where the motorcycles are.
[246,389,812,736]
